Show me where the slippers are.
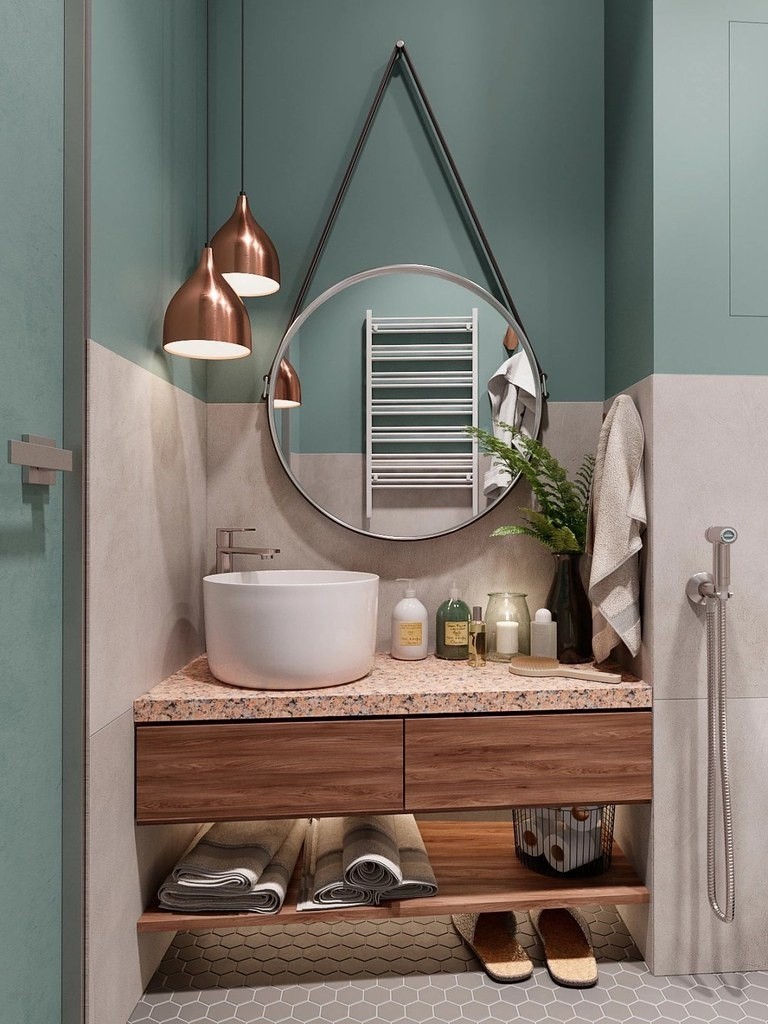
[450,908,599,988]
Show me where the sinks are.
[201,568,381,693]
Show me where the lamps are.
[210,0,279,297]
[163,0,252,361]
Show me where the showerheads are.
[702,524,738,600]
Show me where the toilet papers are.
[560,804,602,831]
[528,804,564,821]
[514,816,561,858]
[541,830,598,874]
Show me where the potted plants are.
[464,418,597,665]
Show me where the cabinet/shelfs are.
[134,710,655,934]
[365,309,478,519]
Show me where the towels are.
[483,348,537,505]
[583,394,649,664]
[147,809,445,916]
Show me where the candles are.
[488,593,530,662]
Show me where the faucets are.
[213,524,284,575]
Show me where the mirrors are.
[267,264,542,541]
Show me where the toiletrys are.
[530,608,561,664]
[435,580,475,661]
[389,575,432,661]
[465,604,487,668]
